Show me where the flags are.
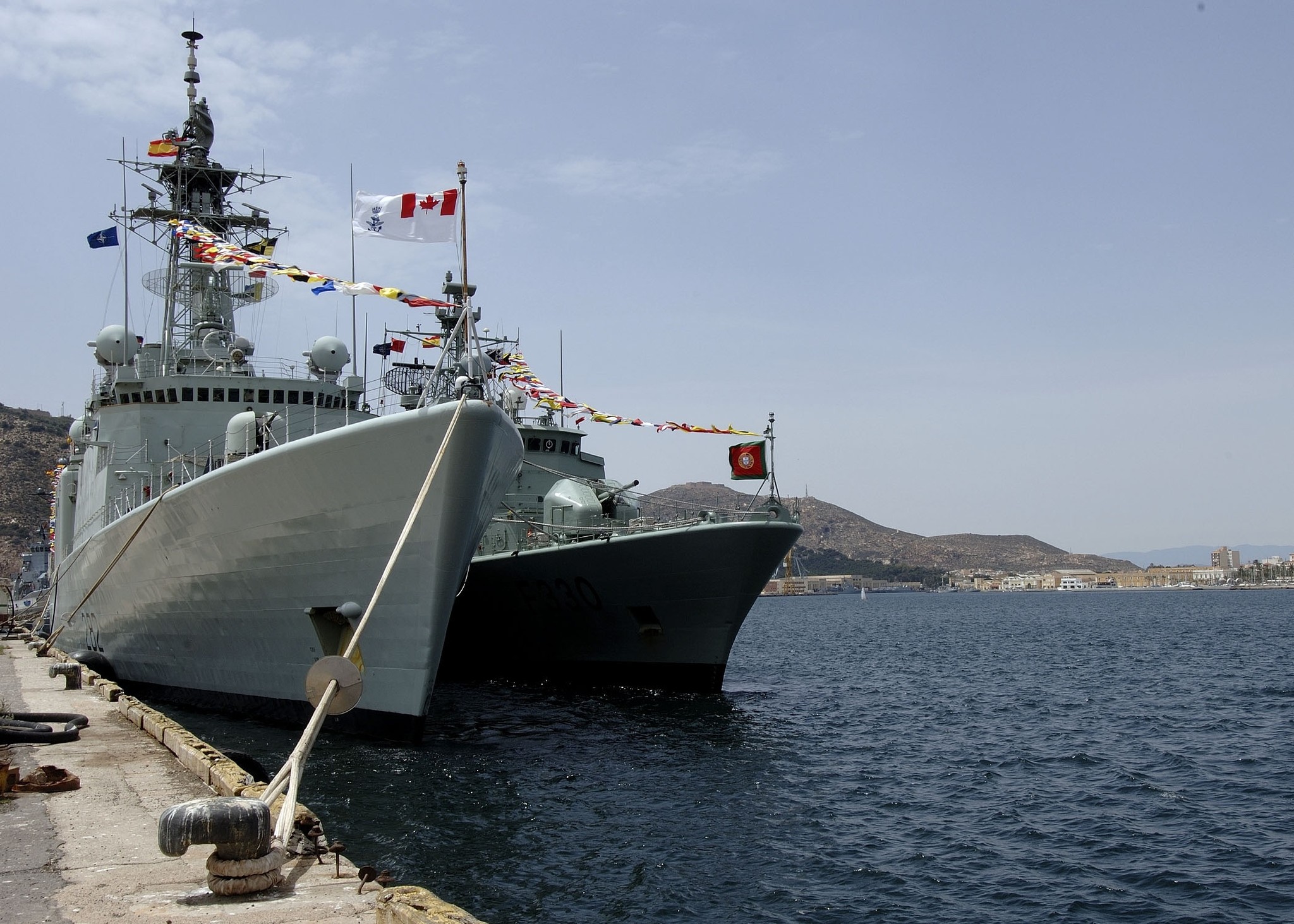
[485,345,771,439]
[170,218,462,316]
[87,225,119,249]
[372,342,391,355]
[353,187,458,242]
[422,335,440,347]
[389,337,406,353]
[148,137,188,157]
[727,438,768,481]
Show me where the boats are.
[7,524,56,630]
[353,159,806,693]
[40,15,527,746]
